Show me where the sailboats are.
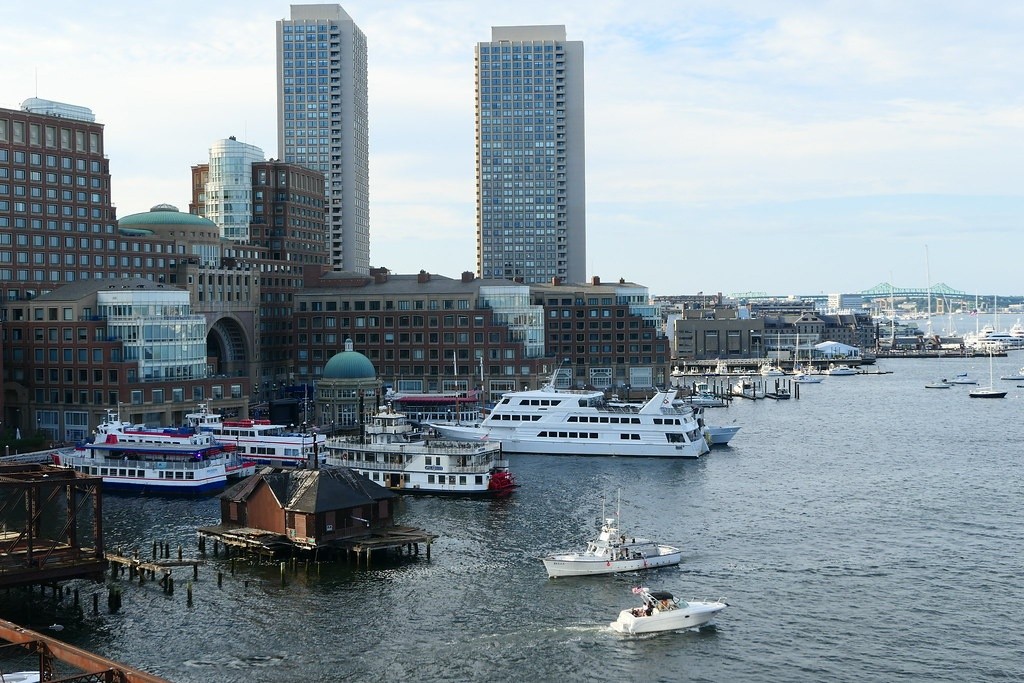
[969,332,1008,399]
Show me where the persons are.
[631,607,638,617]
[657,599,668,610]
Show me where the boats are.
[925,384,951,388]
[529,488,681,578]
[176,402,328,479]
[704,425,742,444]
[49,401,227,494]
[828,365,855,376]
[761,366,784,376]
[860,347,876,365]
[791,340,824,383]
[693,382,712,396]
[609,584,727,635]
[324,390,521,496]
[734,375,765,399]
[672,366,683,377]
[775,388,791,399]
[427,362,711,460]
[880,243,1024,357]
